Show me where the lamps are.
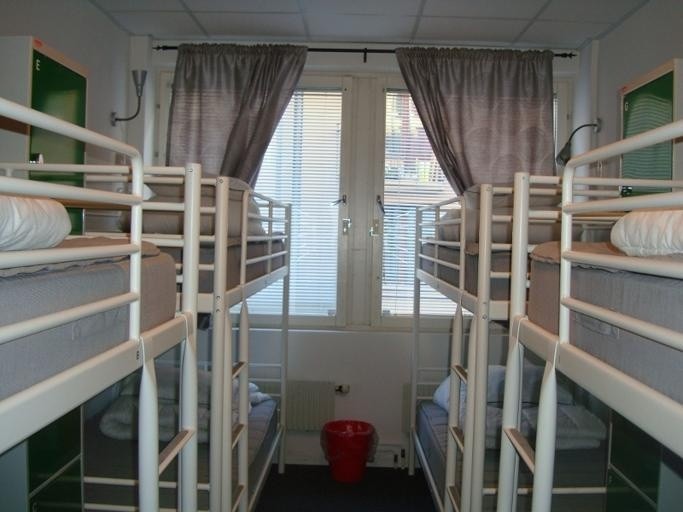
[105,34,154,126]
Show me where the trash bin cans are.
[323,420,374,483]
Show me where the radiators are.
[249,378,347,435]
